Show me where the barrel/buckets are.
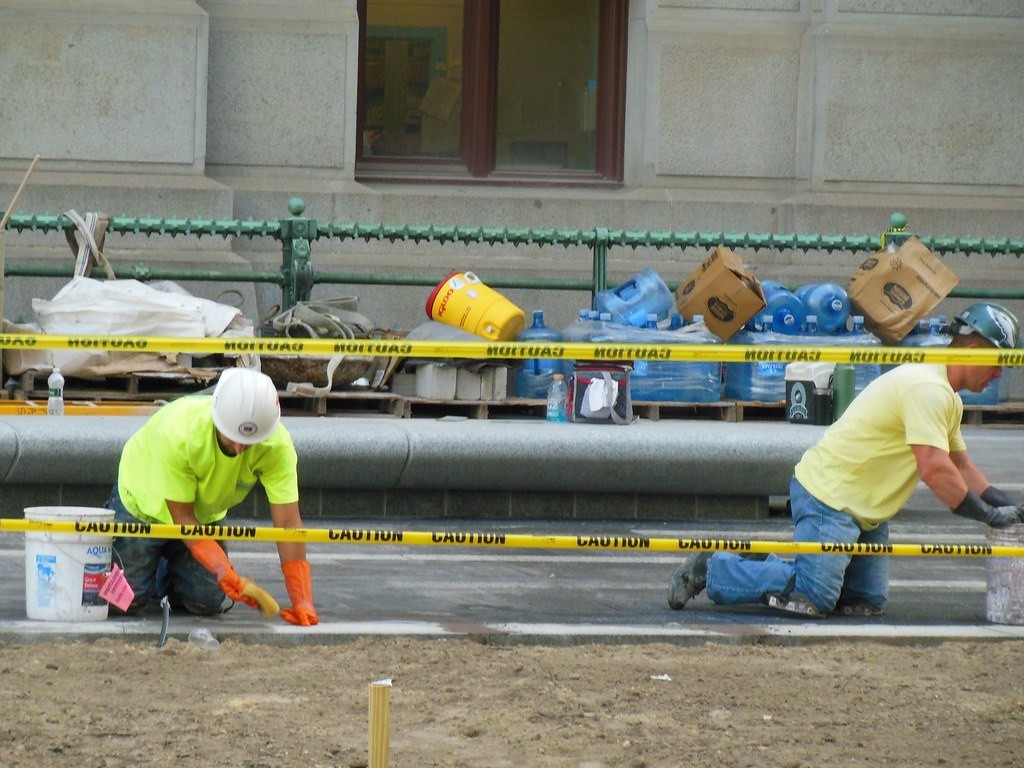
[985,515,1024,626]
[425,272,525,342]
[23,506,116,621]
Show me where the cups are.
[188,627,219,651]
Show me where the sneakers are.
[668,551,715,610]
[739,551,770,561]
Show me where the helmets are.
[210,367,281,443]
[952,302,1018,350]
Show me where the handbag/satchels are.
[567,360,641,425]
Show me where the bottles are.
[547,374,567,423]
[47,368,64,417]
[567,268,722,402]
[515,309,564,399]
[726,281,1009,405]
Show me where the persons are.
[102,367,320,626]
[667,301,1024,620]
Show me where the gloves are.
[192,538,258,608]
[950,492,1024,529]
[979,485,1017,508]
[279,560,320,625]
